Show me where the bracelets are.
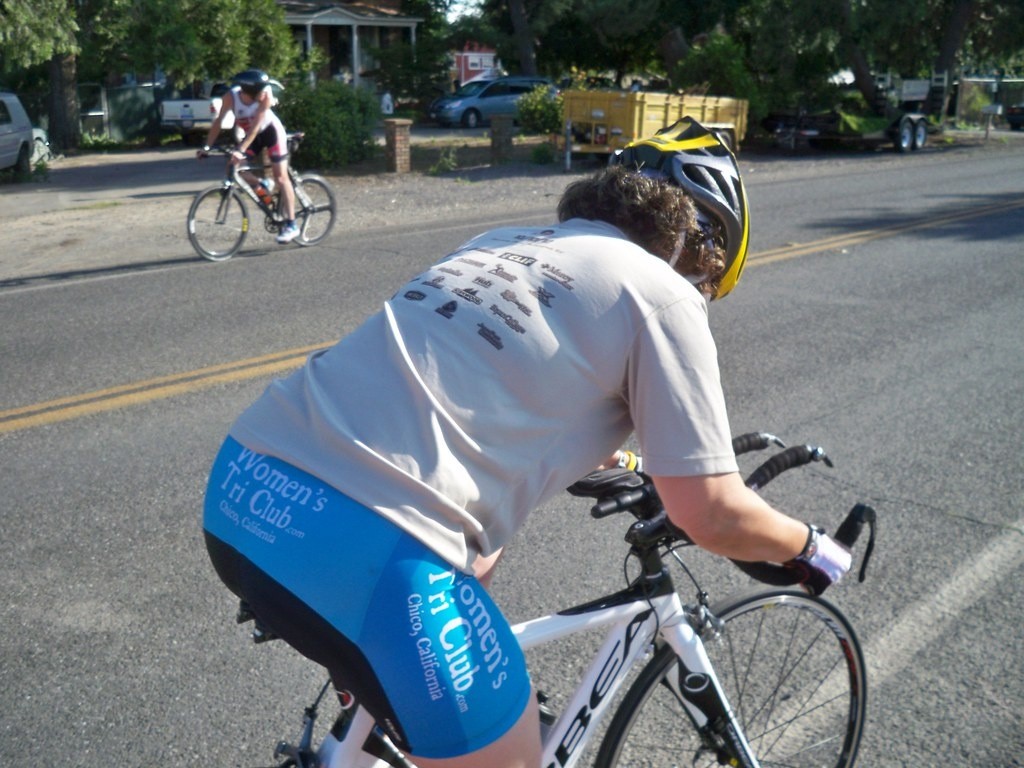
[203,145,210,151]
[624,450,638,471]
[615,452,626,469]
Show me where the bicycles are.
[187,132,339,261]
[237,432,878,767]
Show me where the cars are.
[0,93,34,176]
[427,75,561,128]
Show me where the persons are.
[203,115,852,768]
[196,70,300,243]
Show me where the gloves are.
[782,521,853,599]
[613,450,643,476]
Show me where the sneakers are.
[275,226,301,242]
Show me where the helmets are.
[608,115,751,303]
[228,69,268,86]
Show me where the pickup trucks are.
[159,79,305,157]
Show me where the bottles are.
[253,184,271,205]
[534,691,557,756]
